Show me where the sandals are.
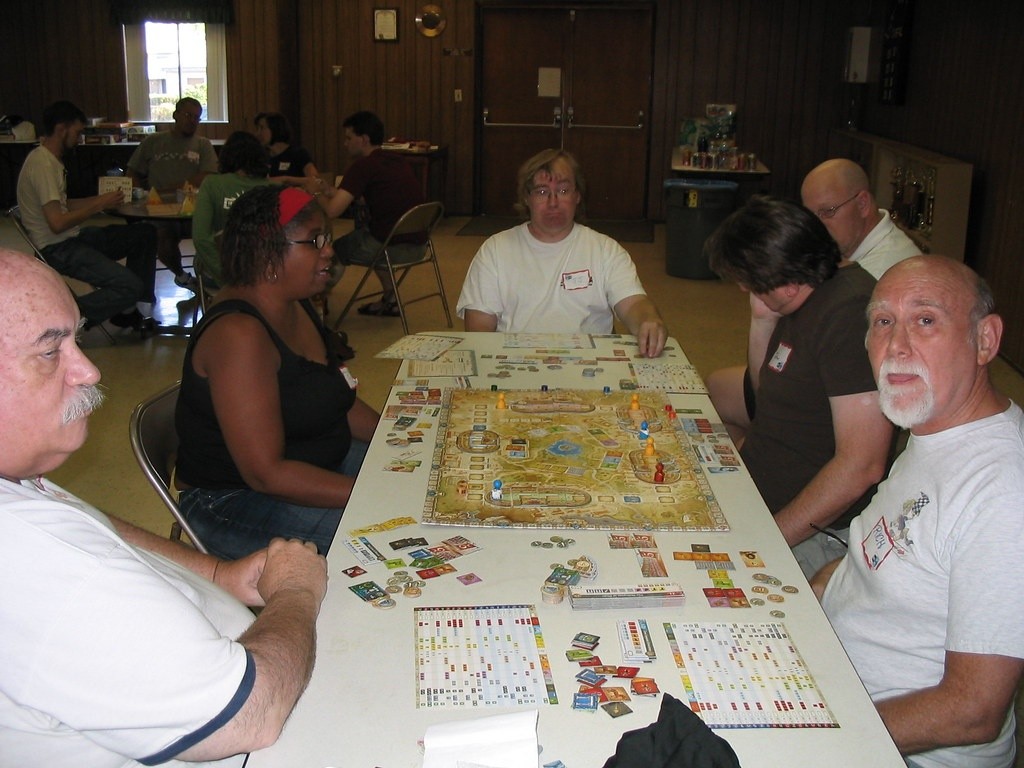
[358,296,402,317]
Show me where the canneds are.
[682,150,756,171]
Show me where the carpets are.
[456,215,656,244]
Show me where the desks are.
[389,142,448,211]
[242,330,909,768]
[0,139,226,216]
[103,192,196,224]
[671,146,772,183]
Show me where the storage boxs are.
[80,117,157,144]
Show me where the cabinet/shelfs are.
[827,126,974,262]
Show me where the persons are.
[176,187,381,560]
[708,195,894,580]
[456,149,669,357]
[810,254,1024,766]
[128,97,219,290]
[193,130,280,289]
[1,246,328,768]
[16,102,157,336]
[255,111,319,188]
[304,111,430,316]
[708,159,921,449]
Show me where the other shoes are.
[175,272,198,295]
[110,306,146,331]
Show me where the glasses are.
[814,193,859,220]
[284,231,332,250]
[178,110,198,121]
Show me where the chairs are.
[129,380,210,555]
[197,230,222,320]
[335,201,454,336]
[7,206,116,344]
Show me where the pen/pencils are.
[116,184,122,192]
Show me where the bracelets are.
[312,192,320,199]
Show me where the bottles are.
[698,136,707,153]
[730,147,738,170]
[719,142,728,167]
[708,141,717,152]
